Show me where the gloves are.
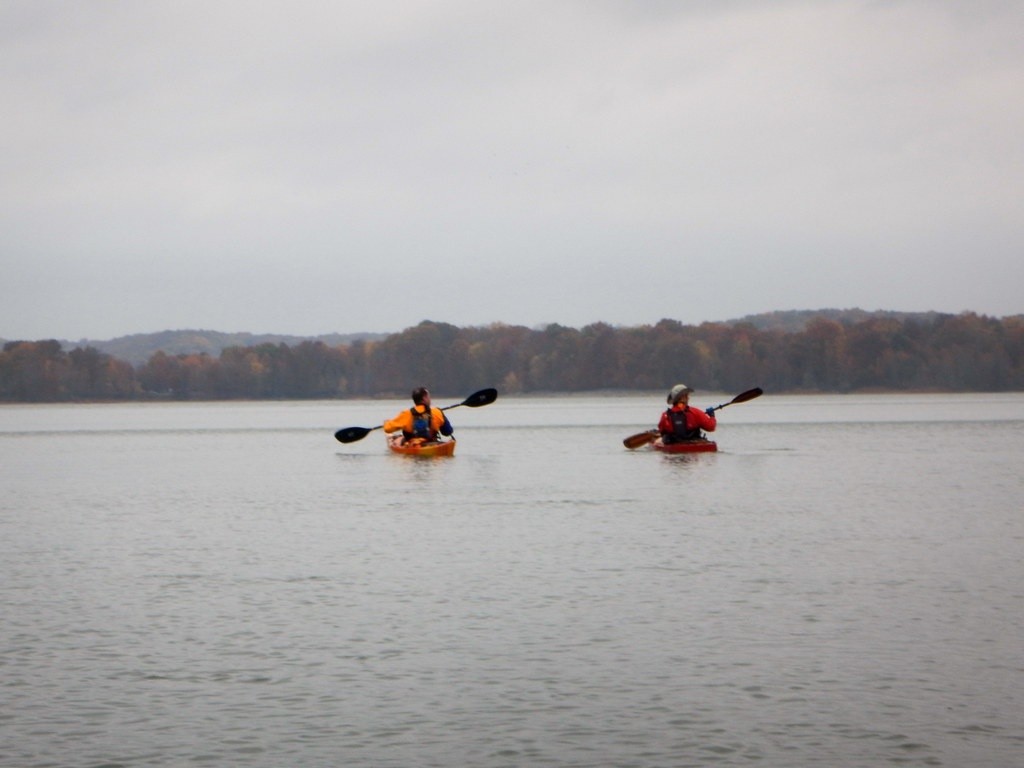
[706,406,715,418]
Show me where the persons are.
[658,384,717,446]
[384,388,454,444]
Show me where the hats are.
[667,384,694,404]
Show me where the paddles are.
[333,385,499,445]
[622,387,763,450]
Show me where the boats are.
[386,433,455,455]
[651,439,718,454]
